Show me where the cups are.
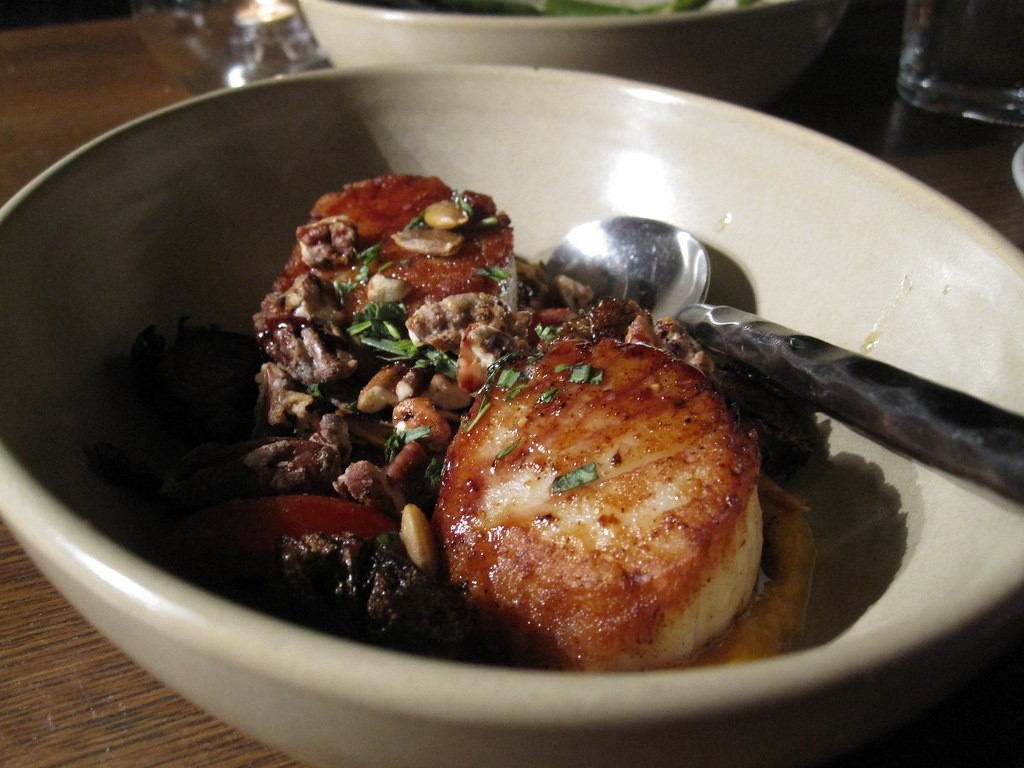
[131,0,333,96]
[895,0,1024,127]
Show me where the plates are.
[1010,141,1024,199]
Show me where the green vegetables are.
[305,194,605,495]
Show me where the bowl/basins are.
[0,65,1024,768]
[299,0,849,111]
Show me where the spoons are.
[542,215,1024,507]
